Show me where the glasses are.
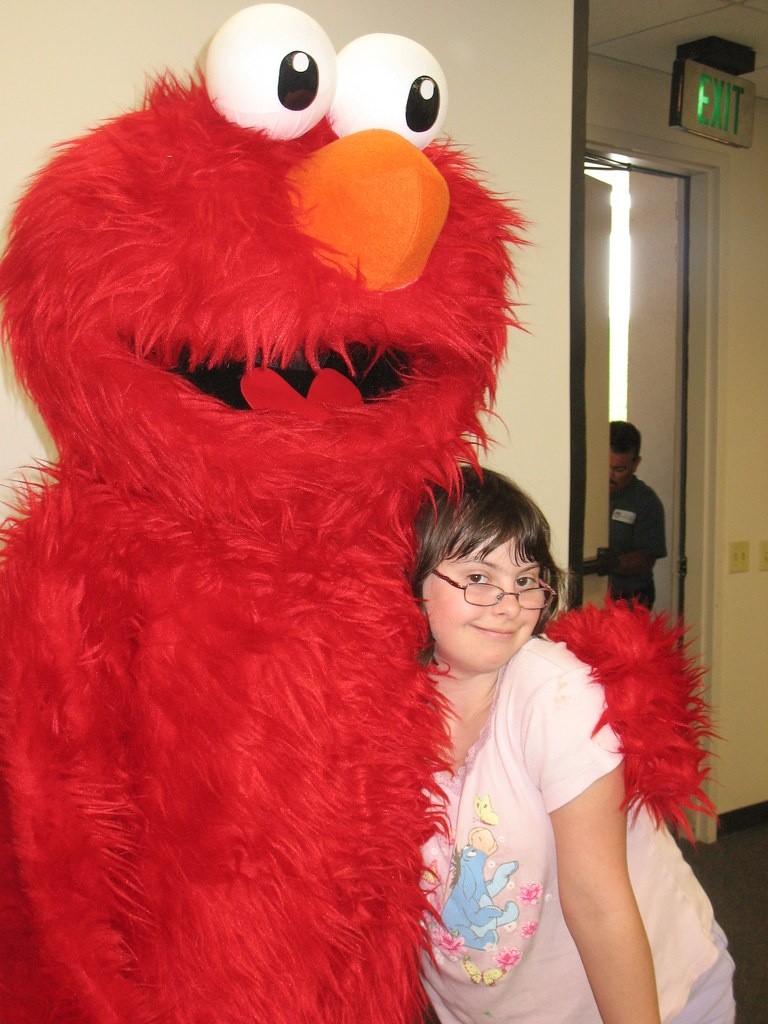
[610,457,636,472]
[432,568,557,610]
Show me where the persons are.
[583,420,668,610]
[404,465,738,1024]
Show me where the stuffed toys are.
[0,1,720,1024]
